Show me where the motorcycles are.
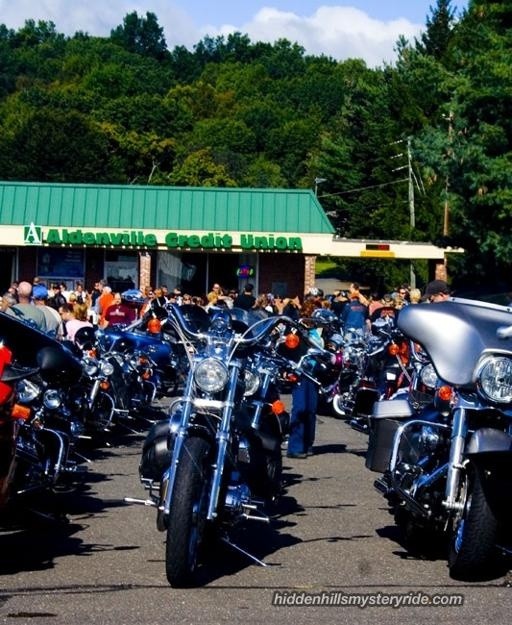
[365,296,511,584]
[1,297,170,524]
[152,297,416,434]
[145,298,344,585]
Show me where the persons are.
[0,272,462,349]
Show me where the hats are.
[33,285,48,297]
[422,280,447,299]
